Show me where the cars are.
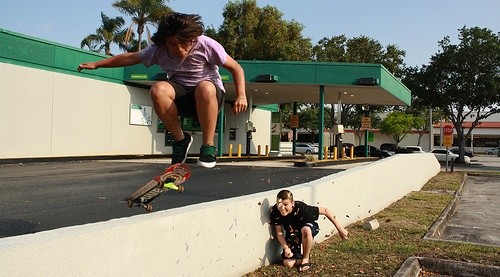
[403,146,426,153]
[486,147,500,155]
[450,148,474,157]
[430,149,472,164]
[379,143,397,152]
[346,145,389,158]
[330,143,356,156]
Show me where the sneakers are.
[172,131,193,165]
[197,146,217,169]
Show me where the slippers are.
[299,262,310,272]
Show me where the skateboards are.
[124,162,190,210]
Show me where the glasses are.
[165,38,198,47]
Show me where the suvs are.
[294,143,318,155]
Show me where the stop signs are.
[442,125,454,135]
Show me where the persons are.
[394,147,412,153]
[77,12,248,168]
[269,190,350,271]
